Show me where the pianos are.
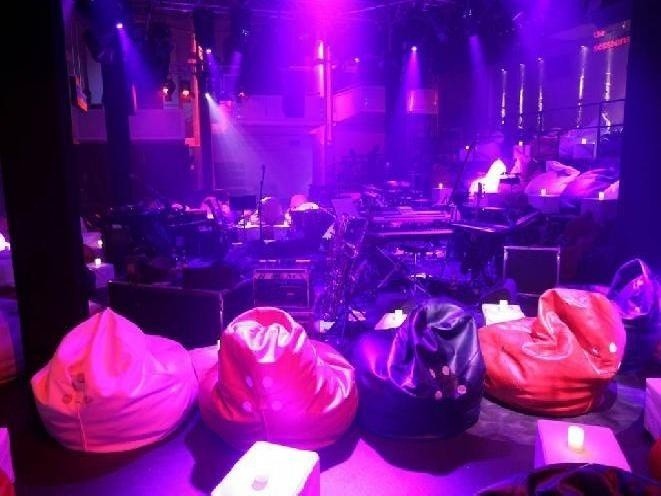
[369,206,462,239]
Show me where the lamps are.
[112,16,125,30]
[161,78,212,102]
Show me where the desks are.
[367,228,453,296]
[369,210,451,228]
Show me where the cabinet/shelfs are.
[253,256,317,312]
[107,276,257,349]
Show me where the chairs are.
[272,225,294,241]
[234,226,261,243]
[560,221,602,280]
[502,244,562,304]
